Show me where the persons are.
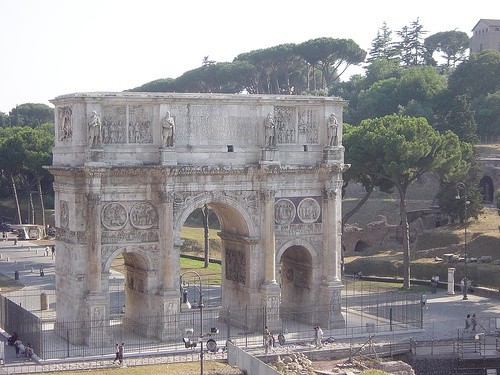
[274,203,318,221]
[121,305,126,313]
[431,272,442,291]
[88,111,103,150]
[102,119,153,143]
[112,342,125,366]
[311,325,325,350]
[59,202,69,228]
[356,271,363,279]
[8,331,34,359]
[461,276,472,298]
[464,313,478,335]
[332,292,341,316]
[263,325,274,347]
[280,127,296,144]
[262,112,279,151]
[418,292,428,311]
[102,206,156,227]
[267,296,277,319]
[165,302,177,328]
[278,333,285,345]
[191,300,204,308]
[1,221,56,276]
[59,108,73,141]
[93,306,103,329]
[161,111,177,149]
[326,113,339,146]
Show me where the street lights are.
[179,270,219,375]
[455,182,470,300]
